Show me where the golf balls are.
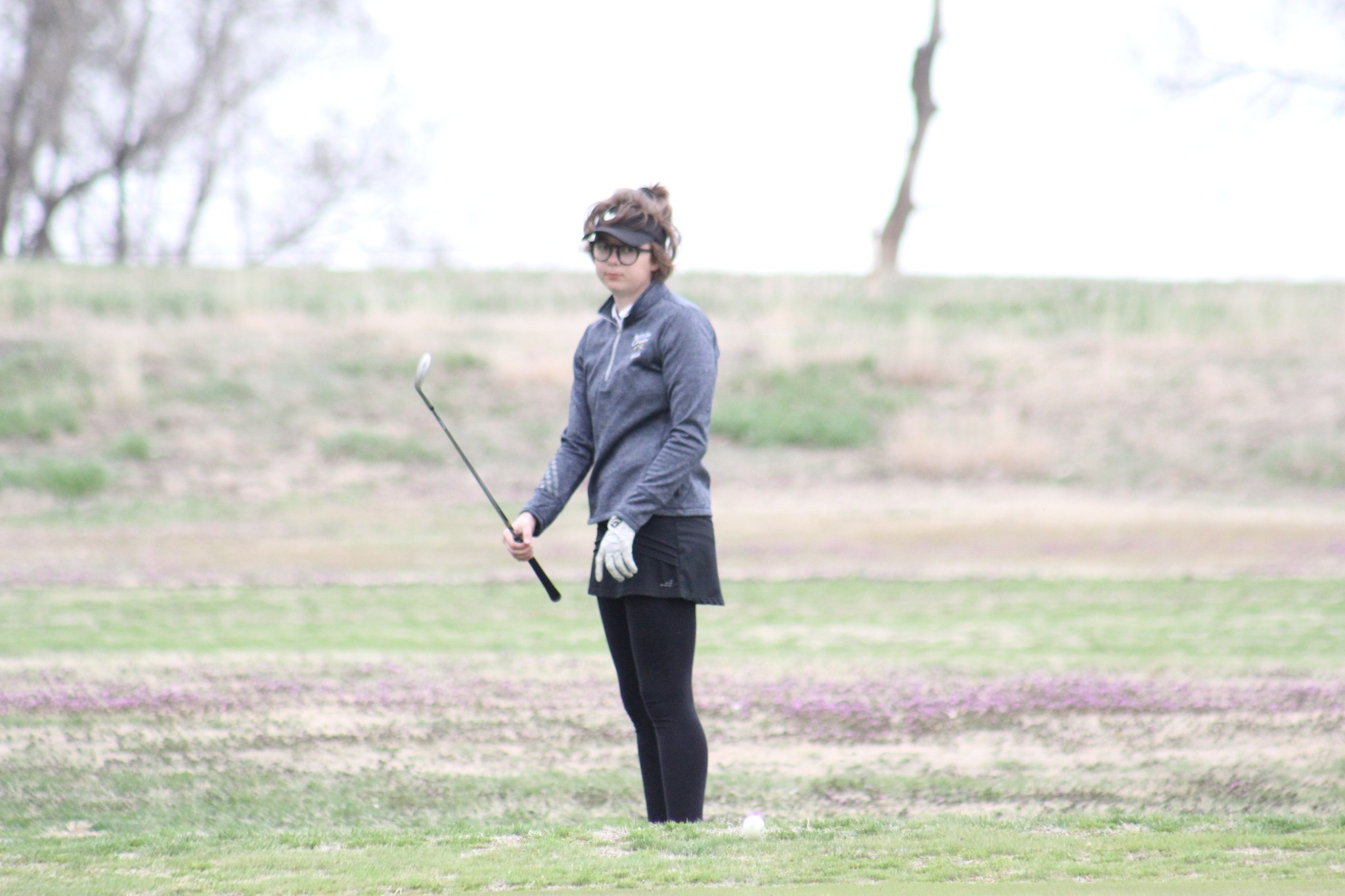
[742,815,767,835]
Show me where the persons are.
[503,185,724,827]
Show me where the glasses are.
[589,241,653,265]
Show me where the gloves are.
[595,515,638,582]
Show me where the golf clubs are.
[413,352,563,602]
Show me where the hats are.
[581,201,668,247]
